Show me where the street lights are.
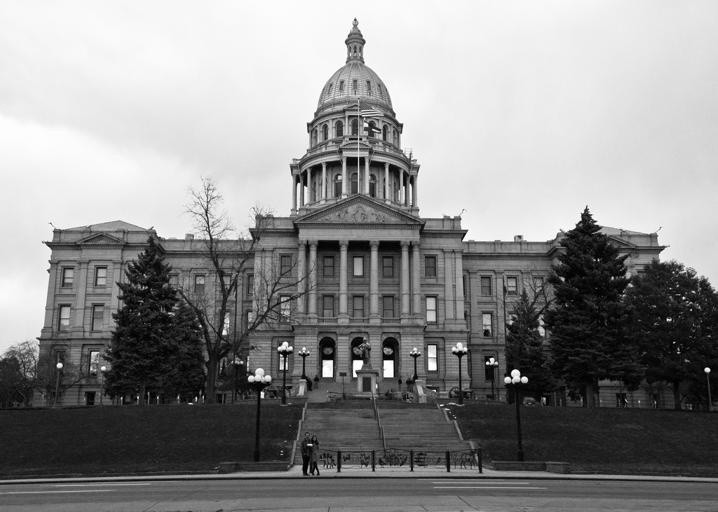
[703,367,712,411]
[276,341,293,407]
[452,342,468,405]
[409,347,421,381]
[485,356,528,460]
[248,367,272,462]
[298,347,310,380]
[54,360,66,407]
[100,366,107,405]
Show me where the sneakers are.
[302,471,321,476]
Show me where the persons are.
[313,374,319,390]
[397,375,402,391]
[311,435,320,475]
[405,377,412,390]
[299,431,313,476]
[357,336,372,365]
